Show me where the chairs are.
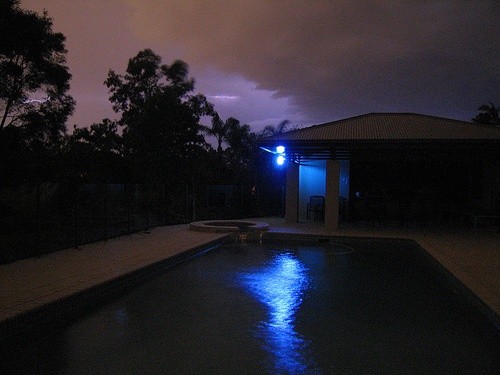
[307,196,327,224]
[338,196,346,226]
